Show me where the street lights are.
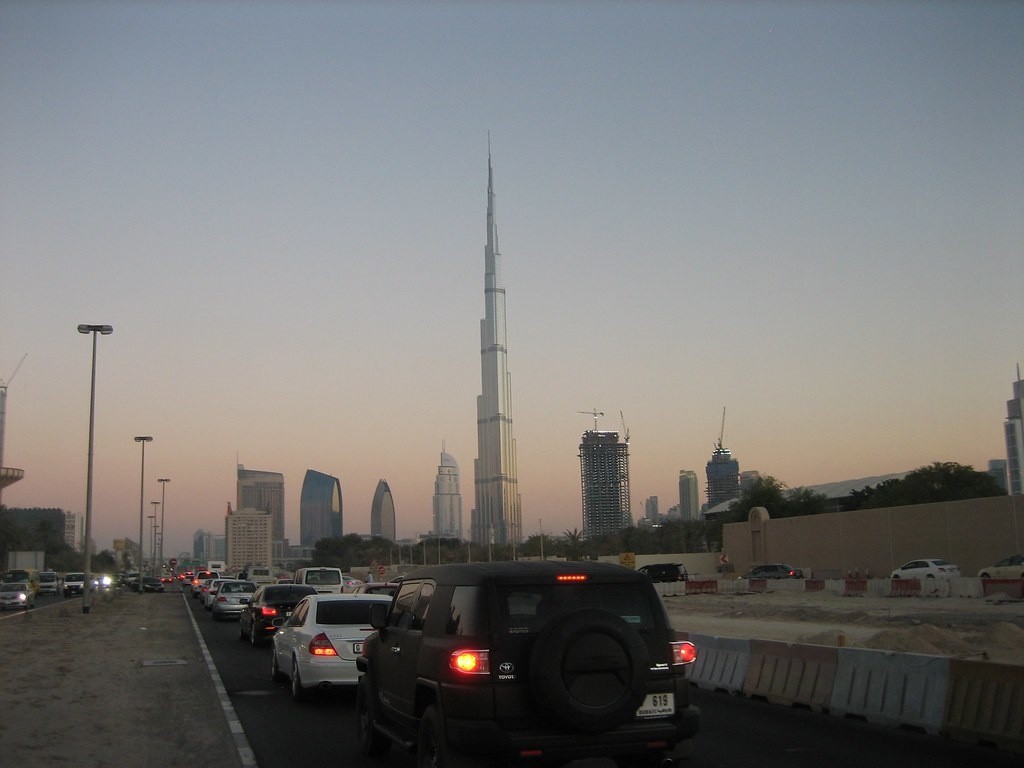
[74,323,115,613]
[156,478,172,559]
[151,500,159,558]
[132,436,154,592]
[147,513,155,557]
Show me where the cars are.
[159,559,235,603]
[211,579,261,619]
[276,578,295,584]
[239,581,318,646]
[635,562,688,586]
[98,571,165,593]
[737,564,805,581]
[0,570,35,611]
[269,591,400,704]
[205,578,241,610]
[891,559,962,579]
[979,554,1024,581]
[341,575,405,596]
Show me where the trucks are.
[238,565,273,584]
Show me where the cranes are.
[579,408,604,430]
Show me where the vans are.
[294,566,344,595]
[36,571,63,595]
[62,572,96,594]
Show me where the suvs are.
[353,559,706,768]
